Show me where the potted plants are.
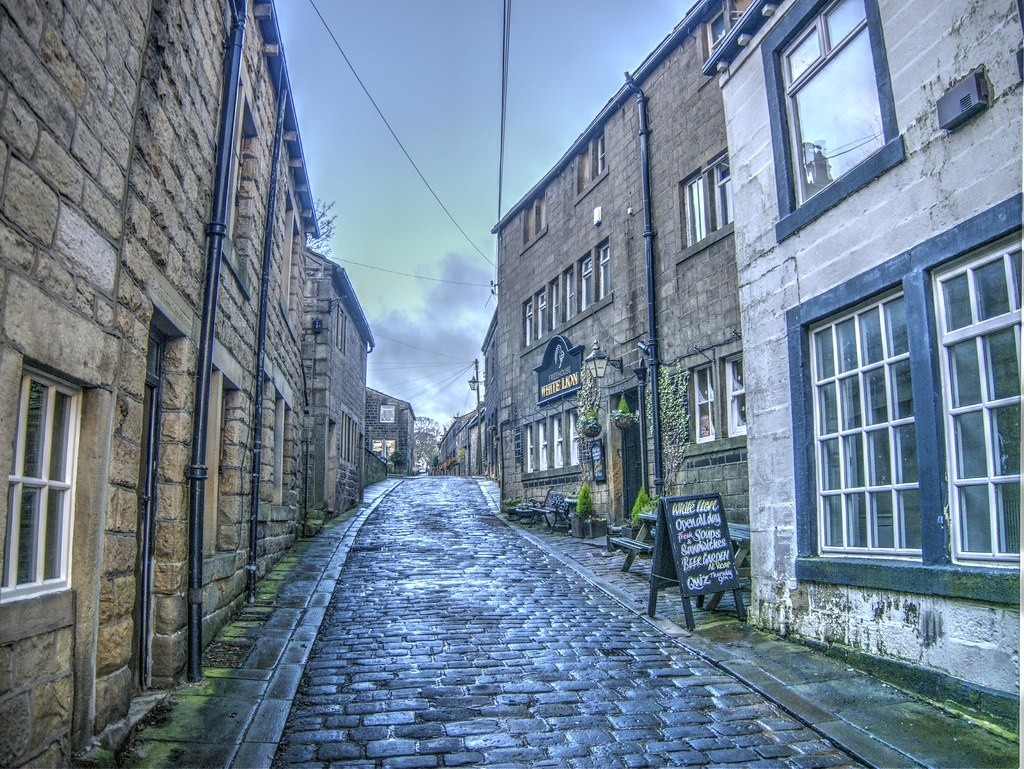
[623,486,653,553]
[569,482,593,538]
[610,393,637,431]
[504,495,533,525]
[581,410,602,438]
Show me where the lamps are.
[586,339,623,379]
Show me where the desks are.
[620,513,750,611]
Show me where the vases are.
[584,521,607,538]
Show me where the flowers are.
[583,512,607,523]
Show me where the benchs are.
[609,536,653,560]
[527,489,576,534]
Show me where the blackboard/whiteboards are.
[651,493,744,599]
[589,438,606,482]
